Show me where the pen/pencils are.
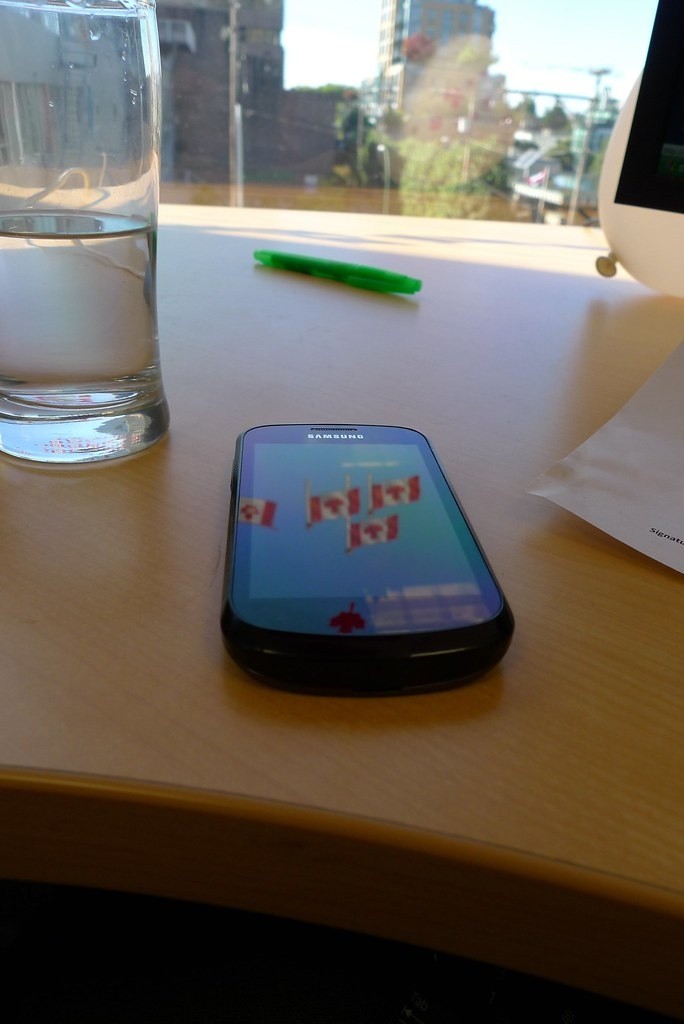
[252,245,423,295]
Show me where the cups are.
[0,0,171,465]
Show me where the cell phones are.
[220,423,514,696]
